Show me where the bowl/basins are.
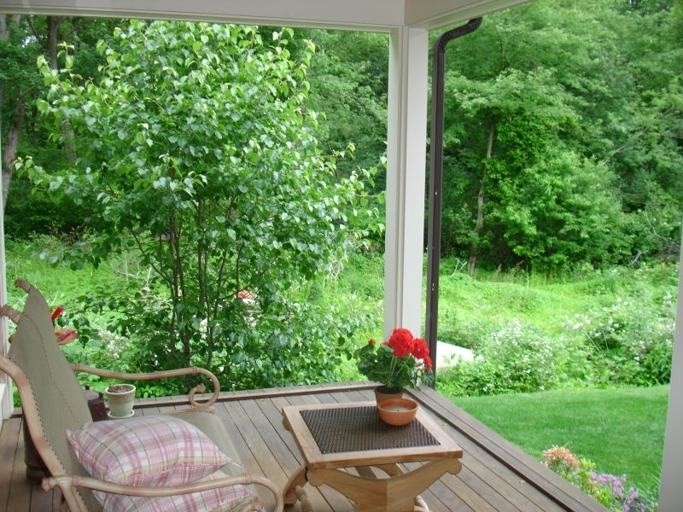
[377,398,418,426]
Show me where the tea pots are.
[82,385,110,421]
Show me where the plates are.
[107,410,134,419]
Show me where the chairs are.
[0,278,283,512]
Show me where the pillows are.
[90,471,257,512]
[66,415,234,486]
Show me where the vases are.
[374,384,402,406]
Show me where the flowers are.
[357,329,433,409]
